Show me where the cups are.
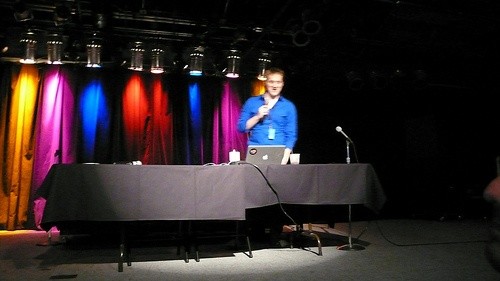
[290,153,300,165]
[229,149,241,163]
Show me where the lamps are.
[149,45,167,74]
[287,8,323,46]
[127,41,145,72]
[12,4,109,69]
[224,48,240,79]
[257,51,273,82]
[186,46,206,77]
[345,70,391,91]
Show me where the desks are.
[36,163,389,272]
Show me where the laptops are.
[245,145,286,164]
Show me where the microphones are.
[264,97,269,119]
[335,126,353,142]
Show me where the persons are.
[237,67,297,247]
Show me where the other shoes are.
[271,231,280,242]
[224,238,246,246]
